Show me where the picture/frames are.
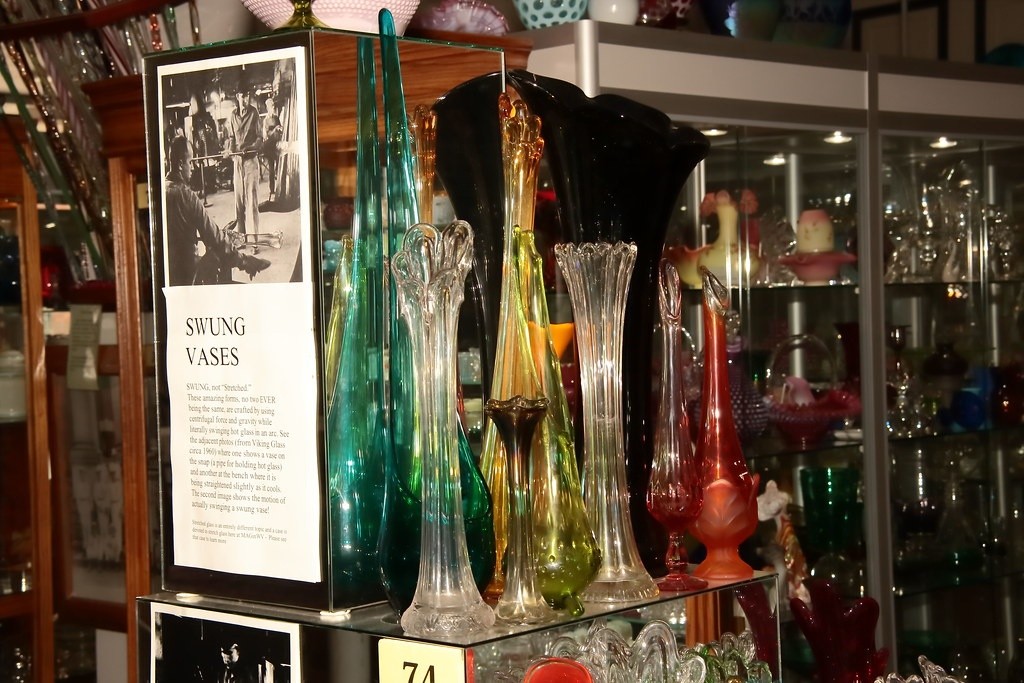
[46,343,160,635]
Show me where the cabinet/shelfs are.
[0,26,532,683]
[506,23,1024,683]
